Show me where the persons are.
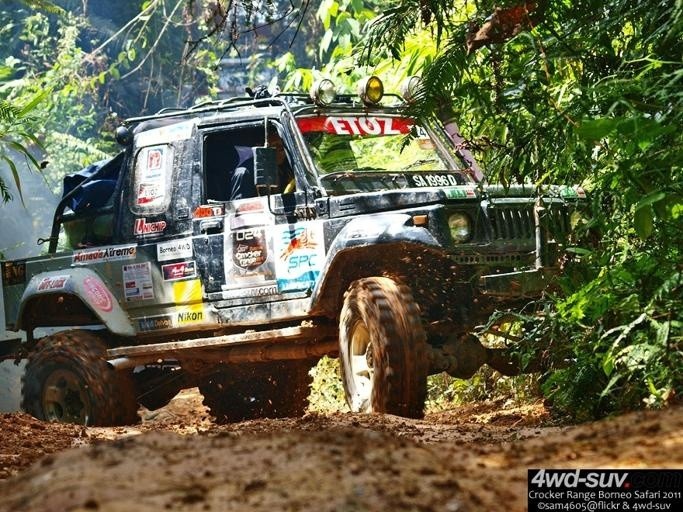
[229,134,355,201]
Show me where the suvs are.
[0,77,605,426]
